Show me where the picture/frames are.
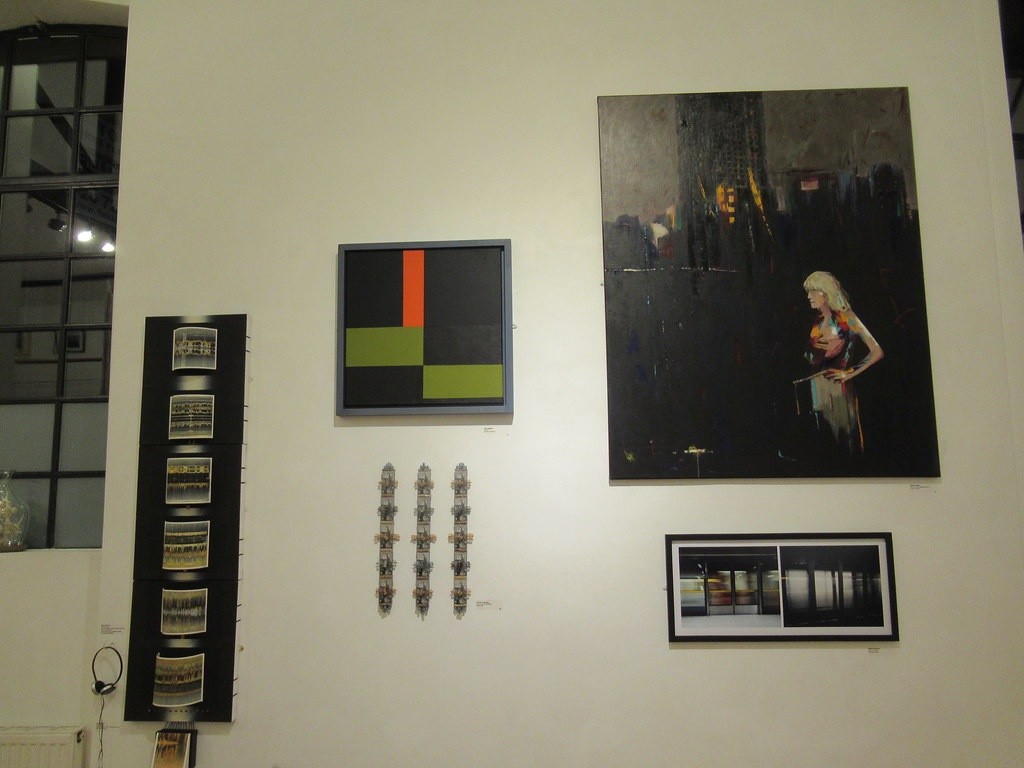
[335,239,513,417]
[666,533,901,642]
[55,330,87,354]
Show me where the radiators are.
[0,725,85,768]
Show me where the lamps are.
[45,211,66,232]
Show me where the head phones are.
[91,646,123,695]
